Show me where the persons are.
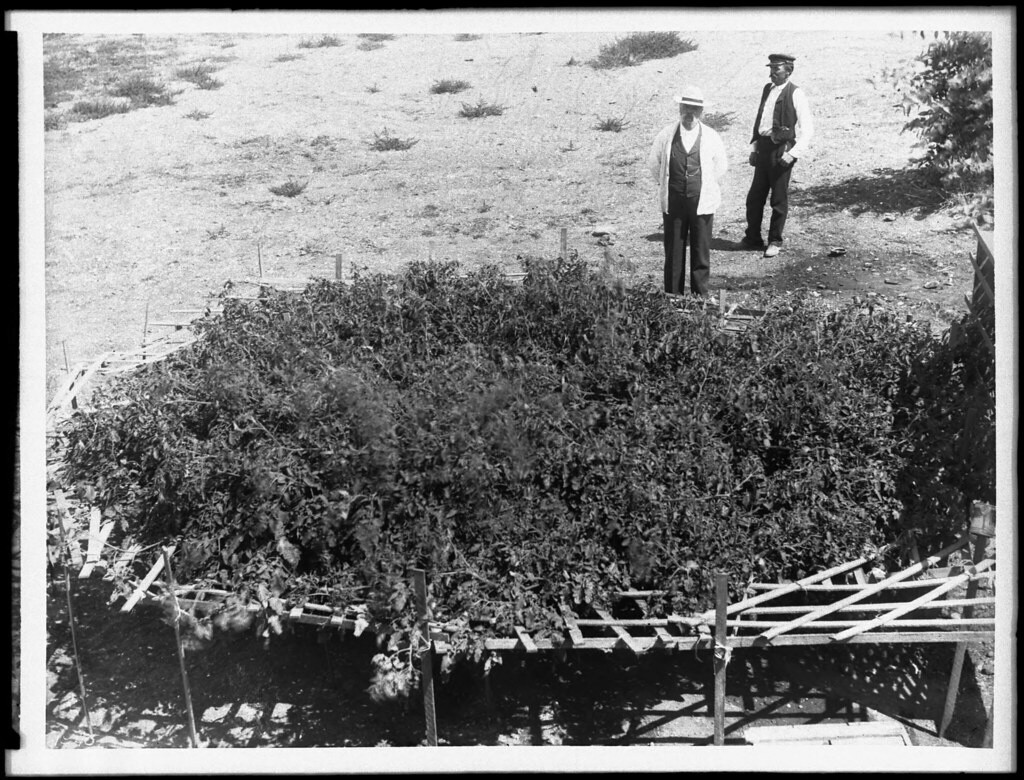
[729,54,811,258]
[647,87,728,298]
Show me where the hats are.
[678,86,707,106]
[765,54,796,66]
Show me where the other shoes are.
[764,245,781,256]
[742,237,764,247]
